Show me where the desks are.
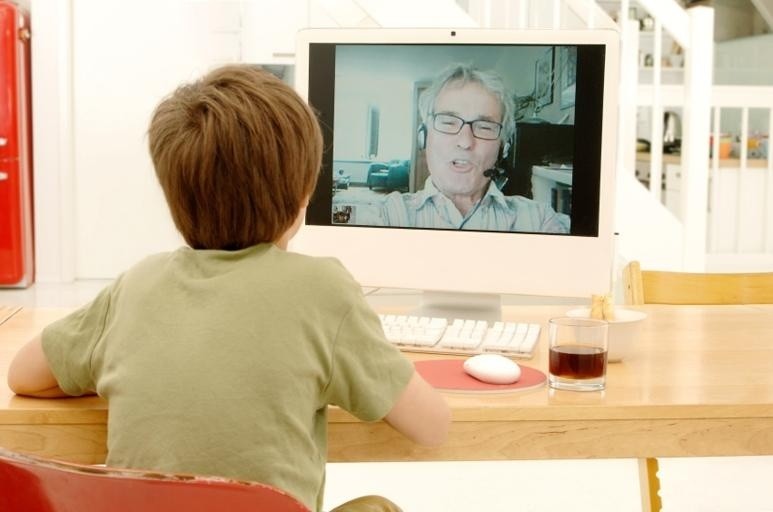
[0,306,773,473]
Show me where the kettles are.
[662,110,682,151]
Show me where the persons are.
[357,62,571,233]
[6,61,455,512]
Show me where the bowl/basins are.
[567,308,648,365]
[709,132,737,160]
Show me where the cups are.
[734,132,770,160]
[546,317,608,392]
[669,53,685,69]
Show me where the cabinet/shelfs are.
[632,31,682,74]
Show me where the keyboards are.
[372,312,543,358]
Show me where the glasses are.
[427,113,502,140]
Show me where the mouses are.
[462,352,520,384]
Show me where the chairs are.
[0,449,310,512]
[367,160,410,193]
[623,260,773,512]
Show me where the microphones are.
[483,166,506,177]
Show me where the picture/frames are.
[534,48,555,110]
[560,47,576,109]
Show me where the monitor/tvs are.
[292,25,613,317]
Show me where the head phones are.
[416,121,512,161]
